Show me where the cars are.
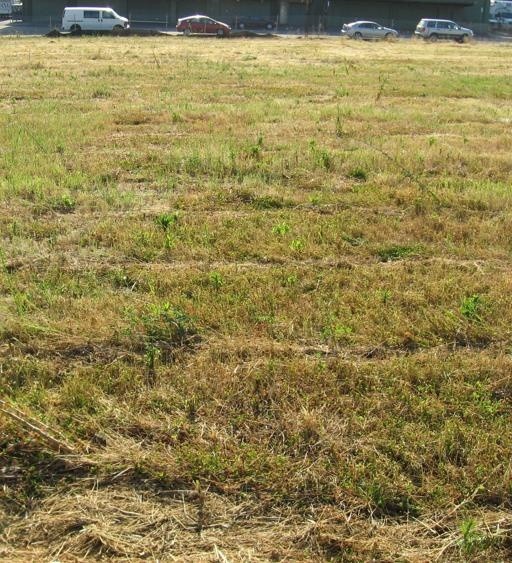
[237,15,275,30]
[341,21,399,41]
[175,15,233,36]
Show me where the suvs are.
[415,18,474,43]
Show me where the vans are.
[60,7,130,33]
